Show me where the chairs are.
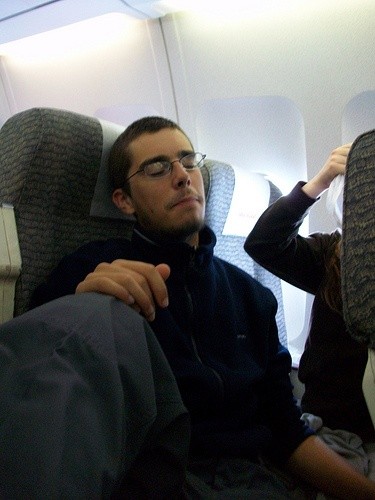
[204,159,288,351]
[340,127,375,430]
[0,107,138,319]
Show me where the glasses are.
[127,152,206,181]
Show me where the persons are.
[242,143,375,449]
[24,117,375,500]
